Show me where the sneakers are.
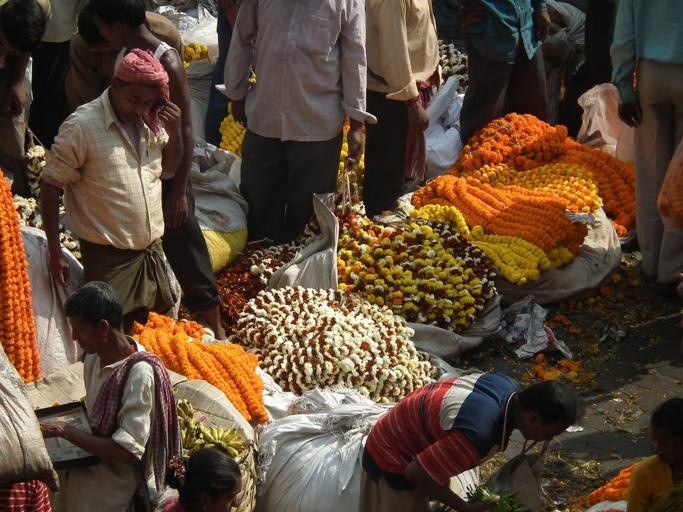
[640,262,683,295]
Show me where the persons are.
[1,0,683,340]
[625,396,682,511]
[33,279,186,512]
[355,368,579,511]
[151,445,243,512]
